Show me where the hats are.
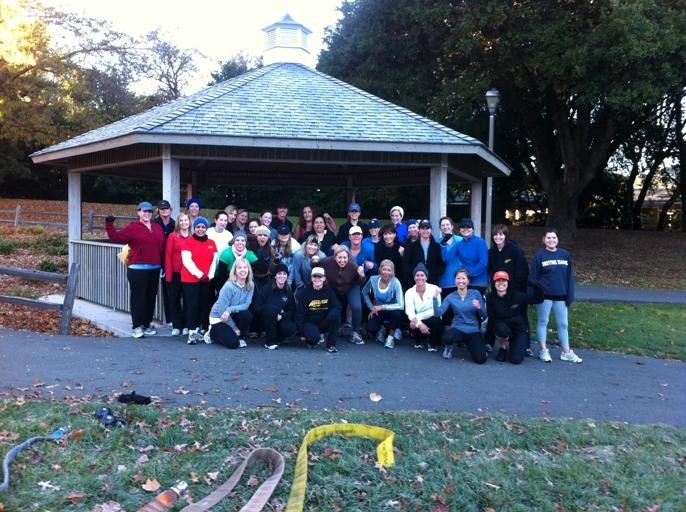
[310,266,326,278]
[418,219,432,230]
[413,262,428,278]
[459,218,475,229]
[271,264,289,278]
[368,219,381,230]
[186,198,201,210]
[492,269,510,283]
[348,225,364,235]
[348,202,361,213]
[137,202,153,214]
[390,206,404,219]
[192,217,209,232]
[276,224,290,236]
[254,226,272,238]
[157,200,171,211]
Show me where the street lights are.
[480,83,505,250]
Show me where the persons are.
[528,229,583,364]
[106,200,534,363]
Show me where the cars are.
[446,193,470,221]
[506,211,545,226]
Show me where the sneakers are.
[442,344,454,360]
[171,327,213,346]
[265,342,279,352]
[537,347,554,364]
[325,345,339,354]
[350,332,365,347]
[237,330,265,350]
[560,349,583,364]
[132,325,157,339]
[413,341,438,353]
[375,328,404,350]
[496,345,508,364]
